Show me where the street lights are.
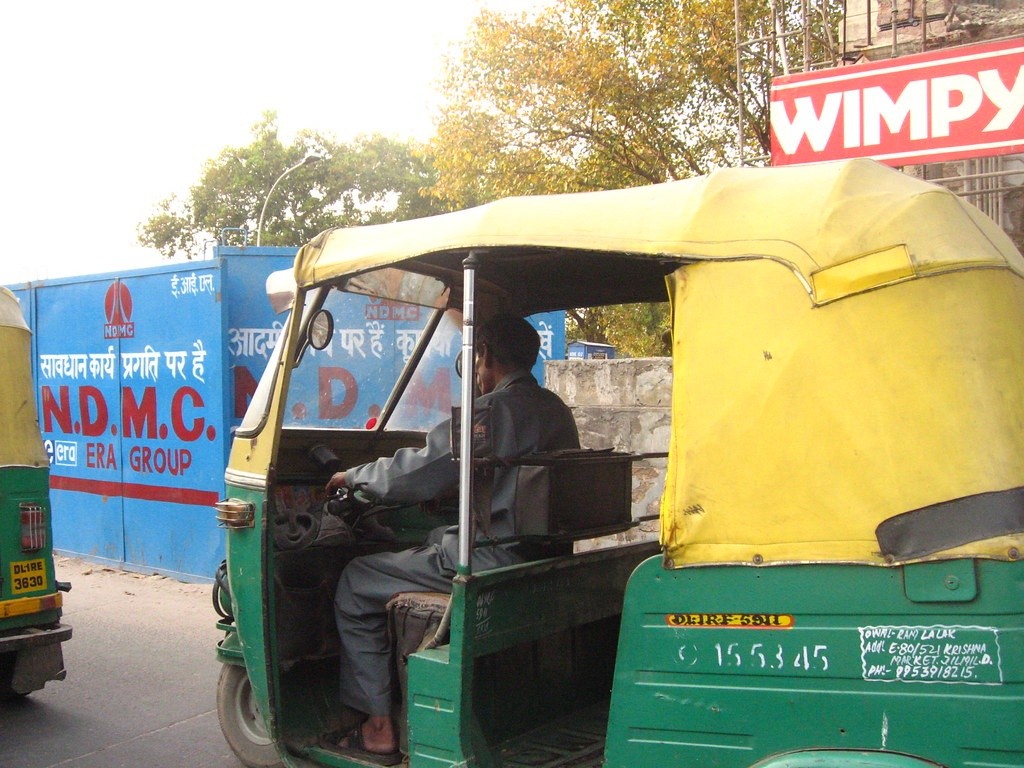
[256,154,323,244]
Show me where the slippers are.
[318,726,403,765]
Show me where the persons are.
[323,310,585,767]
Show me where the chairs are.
[385,444,638,756]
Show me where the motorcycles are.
[0,288,77,689]
[207,161,1022,768]
